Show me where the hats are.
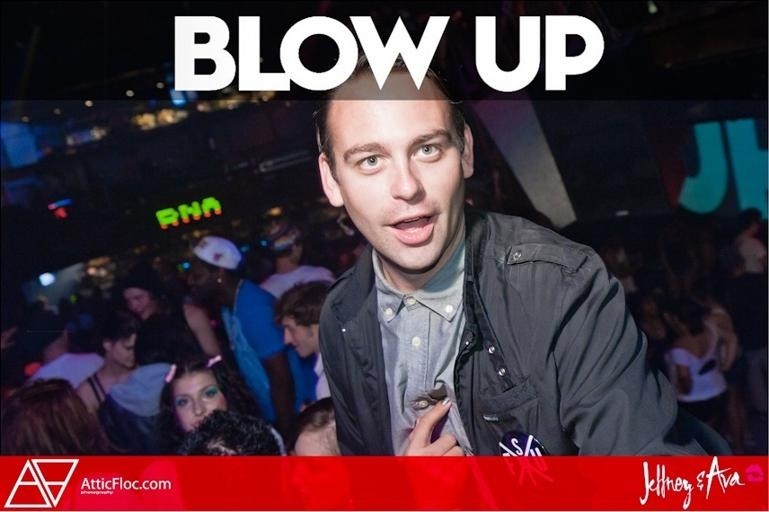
[193,236,242,270]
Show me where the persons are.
[0,210,768,456]
[316,44,731,457]
